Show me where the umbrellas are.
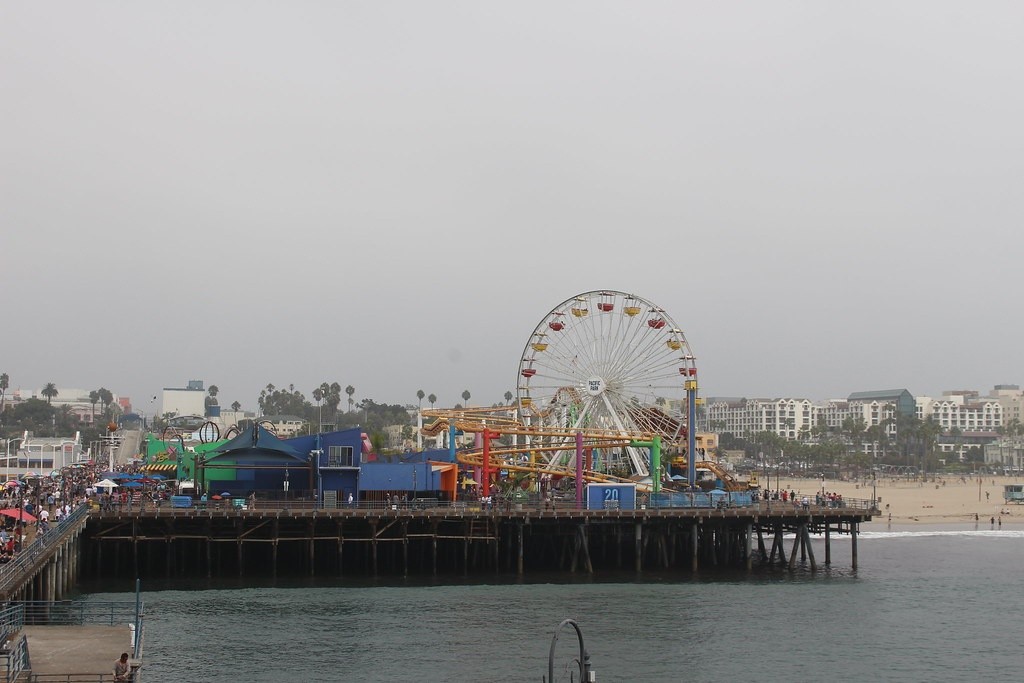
[221,491,231,496]
[0,508,38,537]
[93,479,119,488]
[212,493,222,500]
[148,473,166,480]
[121,480,145,488]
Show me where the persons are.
[0,464,257,564]
[112,653,131,683]
[751,470,1013,526]
[346,492,408,512]
[481,494,556,513]
